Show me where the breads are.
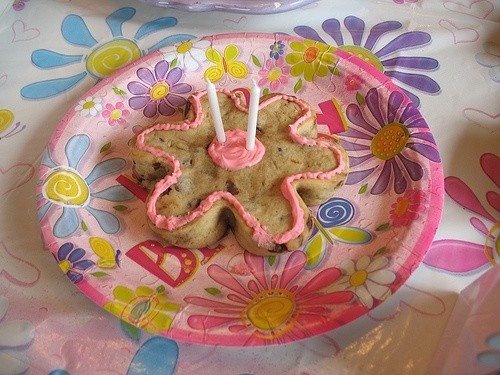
[128,89,349,256]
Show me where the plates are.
[37,31,446,347]
[136,0,321,16]
